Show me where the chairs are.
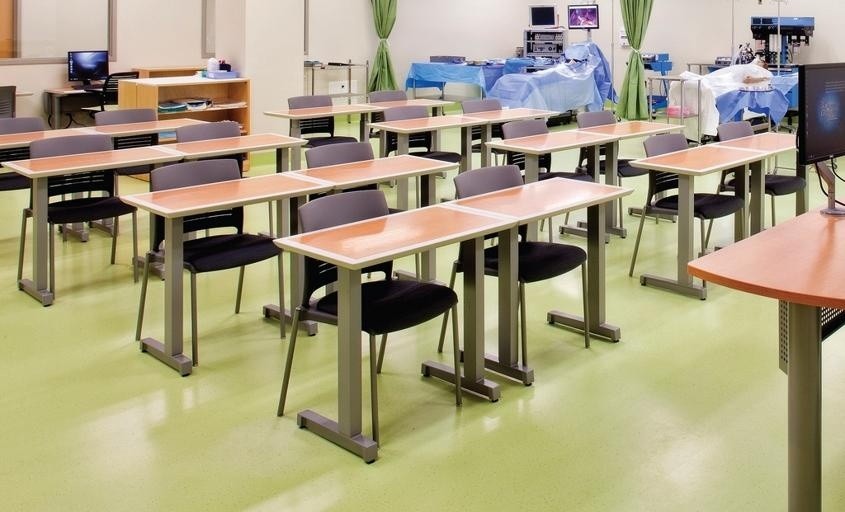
[384,106,461,163]
[18,134,138,300]
[460,100,506,166]
[305,141,406,214]
[438,165,590,365]
[0,85,16,118]
[706,121,806,248]
[277,190,462,447]
[177,120,243,178]
[136,159,287,367]
[0,117,44,190]
[288,95,357,148]
[96,107,157,176]
[80,71,139,119]
[501,119,593,231]
[367,90,408,138]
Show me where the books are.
[158,98,213,113]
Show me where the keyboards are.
[71,84,103,90]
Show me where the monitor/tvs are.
[798,62,845,217]
[68,50,109,85]
[528,3,557,27]
[568,4,599,29]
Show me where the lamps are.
[629,134,744,287]
[564,111,650,238]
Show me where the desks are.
[120,155,459,377]
[366,108,563,210]
[484,120,687,242]
[629,131,799,300]
[771,73,799,132]
[1,132,308,306]
[405,62,504,100]
[272,177,635,463]
[1,118,212,243]
[266,99,457,170]
[43,89,101,129]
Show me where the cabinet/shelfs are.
[686,64,727,74]
[717,87,789,133]
[118,79,251,144]
[505,54,563,73]
[648,75,704,144]
[133,68,207,78]
[304,59,369,123]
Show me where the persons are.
[743,60,773,83]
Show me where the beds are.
[487,42,620,128]
[669,75,771,143]
